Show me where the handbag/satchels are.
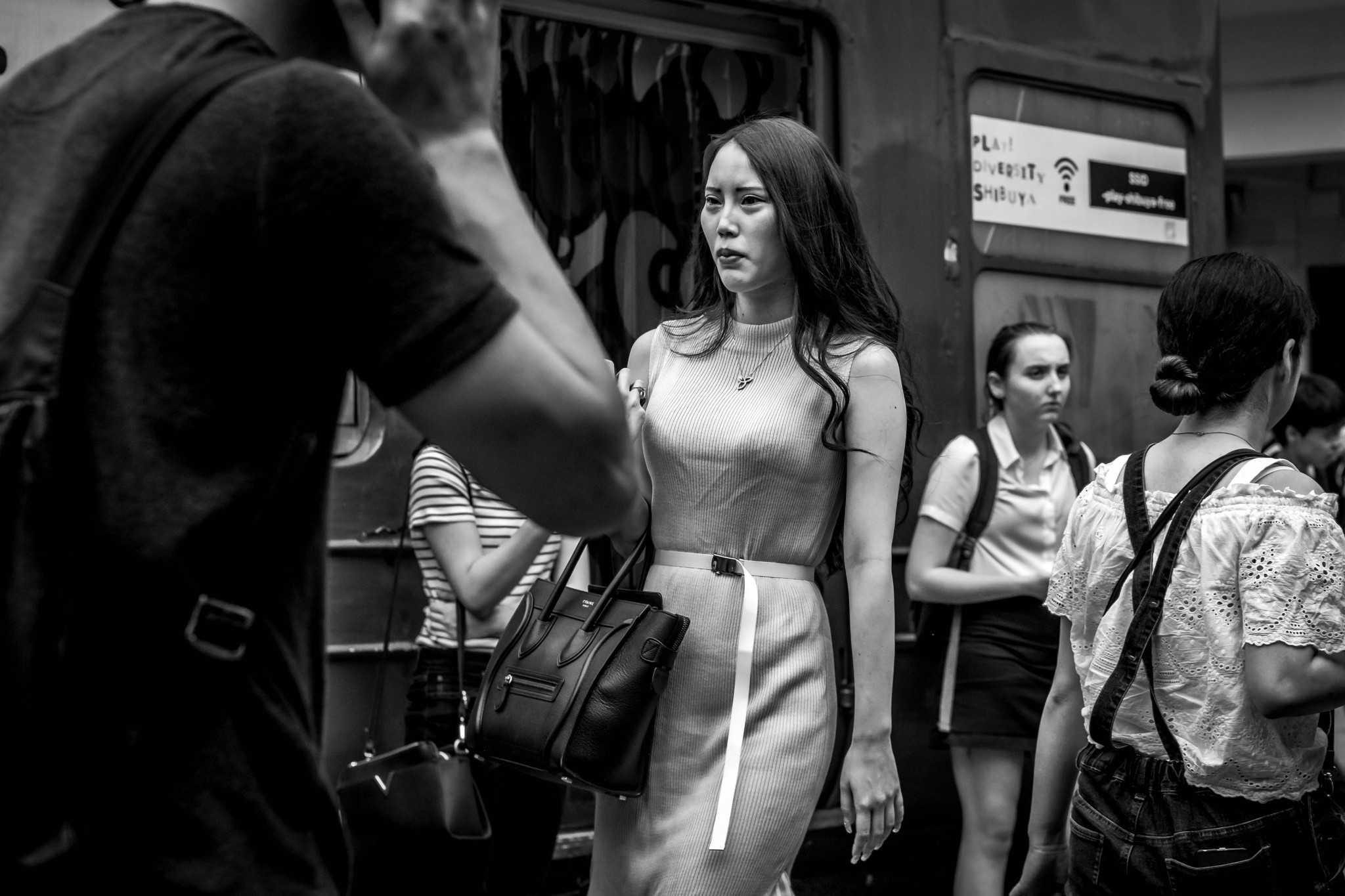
[466,524,690,797]
[335,740,491,856]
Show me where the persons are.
[407,445,591,895]
[1010,254,1345,895]
[0,0,637,896]
[1260,373,1345,479]
[605,118,923,896]
[905,321,1095,896]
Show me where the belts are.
[654,550,815,850]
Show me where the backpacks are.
[0,50,282,896]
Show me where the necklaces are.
[1172,431,1254,449]
[732,308,791,391]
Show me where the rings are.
[630,383,646,407]
